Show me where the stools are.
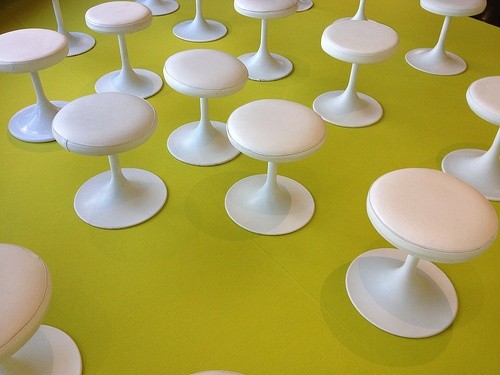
[50,0,95,57]
[0,28,69,144]
[345,168,498,339]
[333,1,381,24]
[84,1,163,100]
[173,0,227,42]
[224,99,328,235]
[135,0,180,16]
[162,49,249,166]
[441,75,500,199]
[0,242,83,375]
[312,20,399,127]
[233,0,299,81]
[51,92,168,231]
[406,1,487,76]
